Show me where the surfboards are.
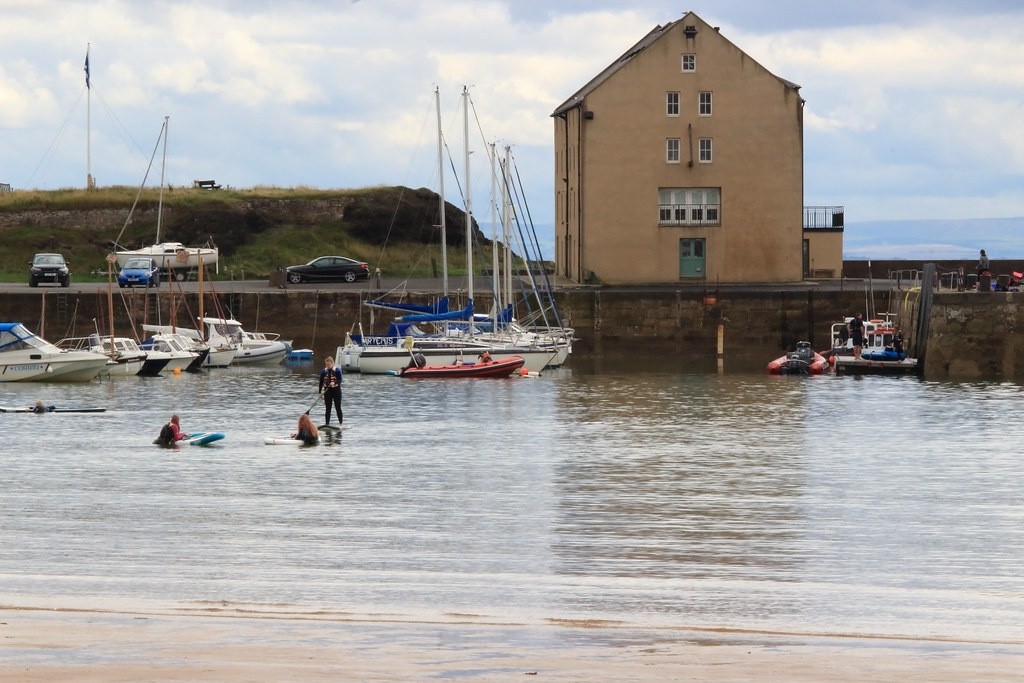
[264,436,323,445]
[173,431,226,446]
[318,423,353,430]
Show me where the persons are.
[319,356,343,428]
[847,312,864,360]
[160,415,187,447]
[976,249,990,282]
[891,325,904,362]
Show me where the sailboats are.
[335,85,576,375]
[113,116,218,269]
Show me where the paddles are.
[298,383,336,431]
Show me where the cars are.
[117,258,160,288]
[286,256,371,284]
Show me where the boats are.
[831,259,918,376]
[768,341,829,375]
[399,347,525,377]
[0,250,293,381]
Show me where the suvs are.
[28,253,70,287]
